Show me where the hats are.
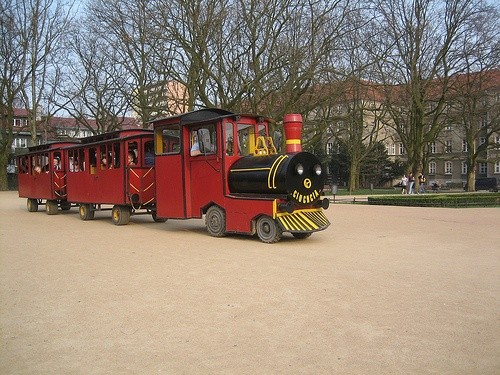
[72,160,78,165]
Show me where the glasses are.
[102,156,106,159]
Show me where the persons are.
[128,151,135,165]
[409,173,415,194]
[416,173,426,194]
[400,174,408,195]
[43,158,60,173]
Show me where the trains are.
[14,108,332,244]
